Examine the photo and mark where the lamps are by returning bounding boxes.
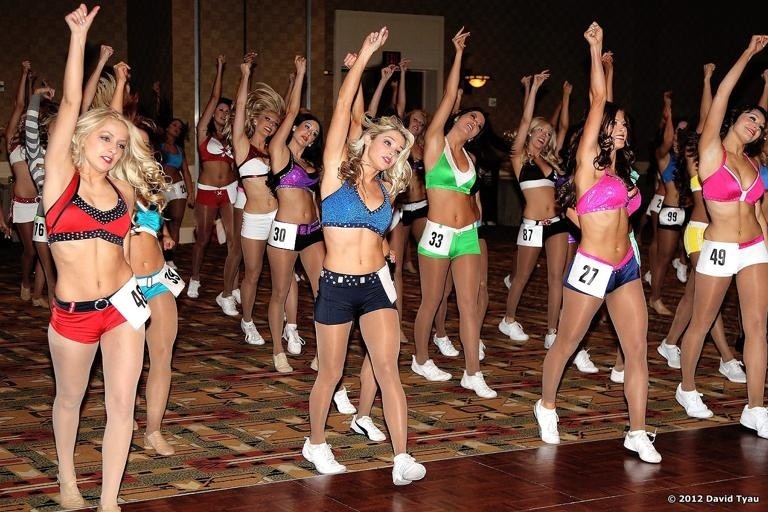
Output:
[465,67,490,89]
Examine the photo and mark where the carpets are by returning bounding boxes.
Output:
[1,236,768,511]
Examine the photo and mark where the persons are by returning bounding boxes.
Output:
[0,3,768,512]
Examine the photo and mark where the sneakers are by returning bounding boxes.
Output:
[460,369,499,400]
[231,288,242,305]
[609,367,624,386]
[644,271,652,284]
[714,356,750,386]
[310,357,320,372]
[504,274,512,290]
[496,316,530,341]
[571,348,600,375]
[298,435,348,477]
[187,277,202,298]
[674,380,716,420]
[399,328,409,344]
[271,349,295,375]
[623,429,666,466]
[350,413,388,444]
[433,333,461,357]
[671,256,689,285]
[141,431,177,458]
[331,385,358,416]
[410,354,452,382]
[239,316,267,346]
[389,451,428,487]
[543,331,558,348]
[477,338,487,362]
[656,337,682,370]
[18,281,31,301]
[166,260,178,271]
[282,322,303,355]
[738,403,768,440]
[530,396,565,446]
[646,297,672,317]
[57,473,85,509]
[30,294,49,310]
[216,290,240,316]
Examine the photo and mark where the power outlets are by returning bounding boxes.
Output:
[488,98,497,107]
